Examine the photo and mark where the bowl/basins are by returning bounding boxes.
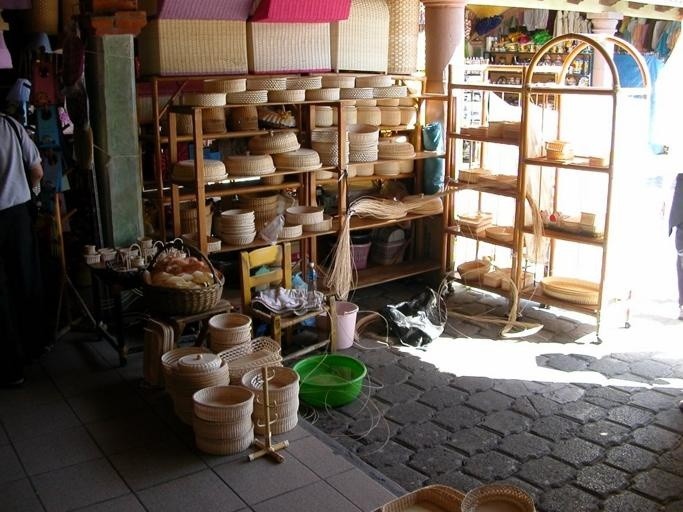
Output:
[292,355,366,408]
[330,229,372,244]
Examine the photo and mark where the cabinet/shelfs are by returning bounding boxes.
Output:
[447,32,651,343]
[145,28,458,318]
[482,43,594,106]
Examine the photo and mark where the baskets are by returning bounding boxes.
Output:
[460,121,521,139]
[546,141,608,167]
[456,210,514,241]
[540,276,599,305]
[560,212,594,237]
[83,235,158,266]
[140,244,225,317]
[171,75,416,182]
[160,312,300,456]
[457,260,534,292]
[169,194,335,252]
[458,167,517,190]
[370,483,537,512]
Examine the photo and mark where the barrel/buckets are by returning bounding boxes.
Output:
[319,300,359,350]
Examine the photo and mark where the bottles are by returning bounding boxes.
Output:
[543,40,589,76]
[316,184,324,207]
[498,56,506,65]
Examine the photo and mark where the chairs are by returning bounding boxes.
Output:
[238,242,336,363]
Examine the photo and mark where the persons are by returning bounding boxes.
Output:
[667,172,682,319]
[1,112,51,386]
